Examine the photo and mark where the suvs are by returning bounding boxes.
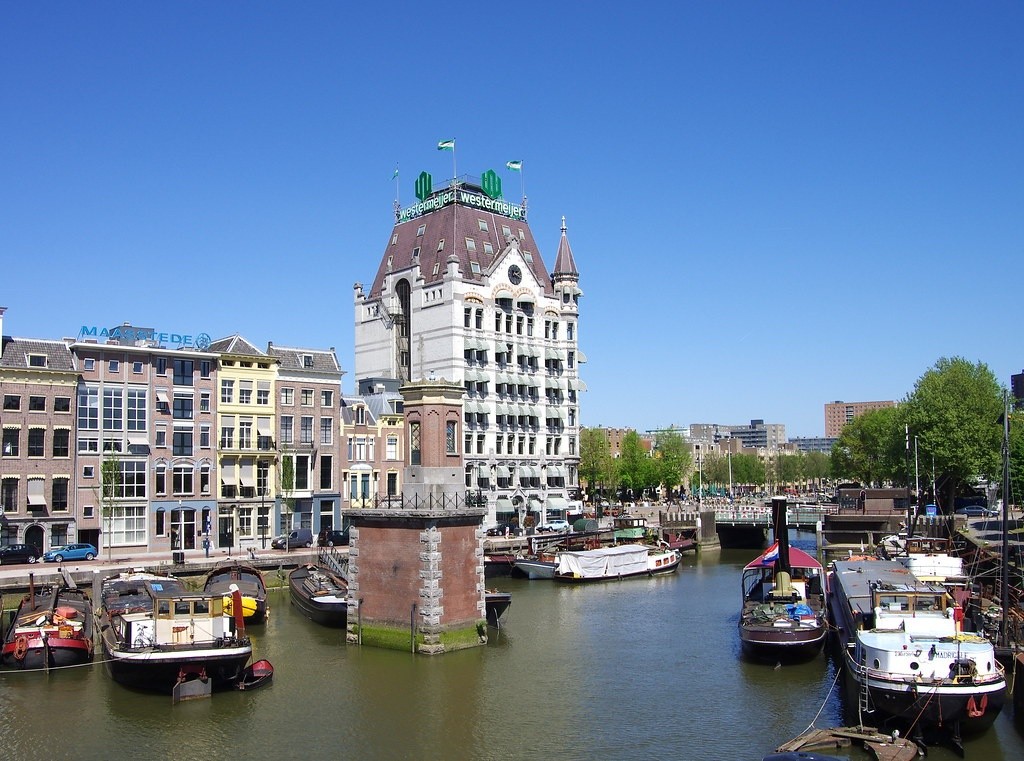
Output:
[544,520,569,532]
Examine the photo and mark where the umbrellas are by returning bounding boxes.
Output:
[690,481,725,499]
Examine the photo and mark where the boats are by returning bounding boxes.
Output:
[237,659,274,692]
[738,496,830,663]
[204,560,267,625]
[289,562,348,628]
[483,537,682,583]
[825,389,1024,759]
[94,561,252,693]
[1,572,94,674]
[485,587,512,629]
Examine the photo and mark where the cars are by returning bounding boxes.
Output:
[317,529,349,547]
[486,523,515,536]
[955,506,999,517]
[0,544,40,565]
[43,543,97,562]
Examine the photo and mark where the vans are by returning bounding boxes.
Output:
[271,529,313,549]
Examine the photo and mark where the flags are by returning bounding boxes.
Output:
[507,160,521,171]
[437,139,453,150]
[762,542,779,565]
[393,166,399,176]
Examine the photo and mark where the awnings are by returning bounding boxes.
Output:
[519,466,532,478]
[529,467,542,477]
[480,466,492,478]
[556,466,566,477]
[529,500,542,512]
[546,466,560,477]
[546,497,569,510]
[496,500,514,512]
[27,495,47,506]
[221,477,237,486]
[240,477,256,487]
[496,466,510,478]
